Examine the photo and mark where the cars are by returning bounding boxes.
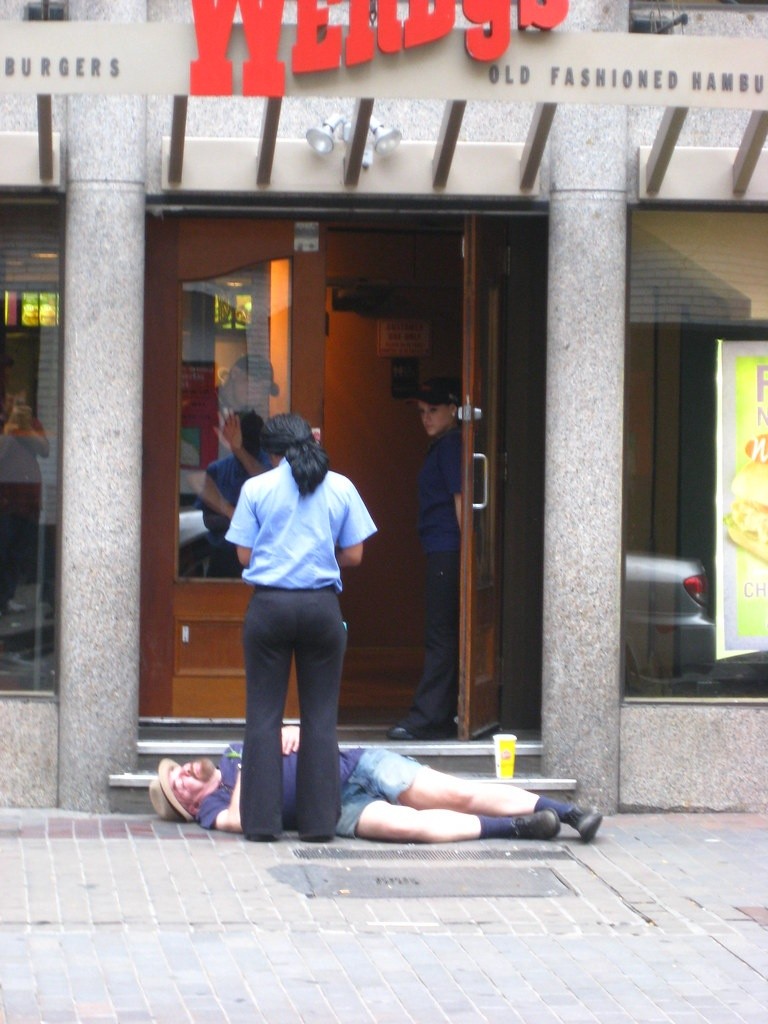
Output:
[624,550,716,679]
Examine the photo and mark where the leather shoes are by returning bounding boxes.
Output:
[564,806,602,842]
[513,808,560,840]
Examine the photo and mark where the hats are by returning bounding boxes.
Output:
[409,377,462,407]
[149,757,194,823]
[235,354,279,397]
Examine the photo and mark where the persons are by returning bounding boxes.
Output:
[0,373,50,612]
[149,726,602,843]
[180,356,280,520]
[386,378,463,738]
[194,410,273,579]
[225,412,377,843]
